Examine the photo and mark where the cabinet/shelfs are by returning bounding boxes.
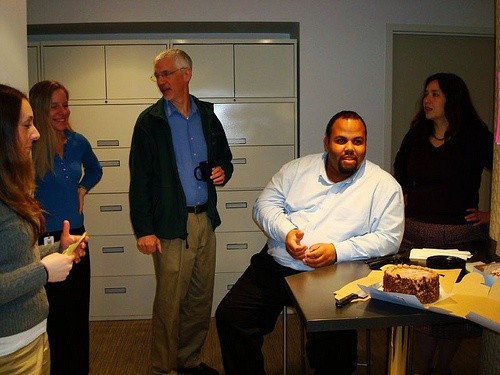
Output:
[168,37,300,320]
[27,38,170,321]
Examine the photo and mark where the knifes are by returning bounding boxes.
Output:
[334,282,382,308]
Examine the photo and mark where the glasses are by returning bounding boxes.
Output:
[150,67,189,82]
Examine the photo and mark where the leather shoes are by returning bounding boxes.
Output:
[177,361,221,375]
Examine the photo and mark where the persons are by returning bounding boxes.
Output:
[214,109,407,375]
[0,82,91,374]
[127,48,235,374]
[29,80,104,375]
[393,72,494,375]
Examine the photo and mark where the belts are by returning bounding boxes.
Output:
[186,203,208,215]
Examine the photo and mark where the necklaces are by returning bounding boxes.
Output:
[432,134,445,141]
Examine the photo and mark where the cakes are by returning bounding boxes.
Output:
[382,264,439,304]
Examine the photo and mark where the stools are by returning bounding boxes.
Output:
[283,306,371,375]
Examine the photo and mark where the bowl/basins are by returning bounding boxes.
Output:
[425,255,465,283]
